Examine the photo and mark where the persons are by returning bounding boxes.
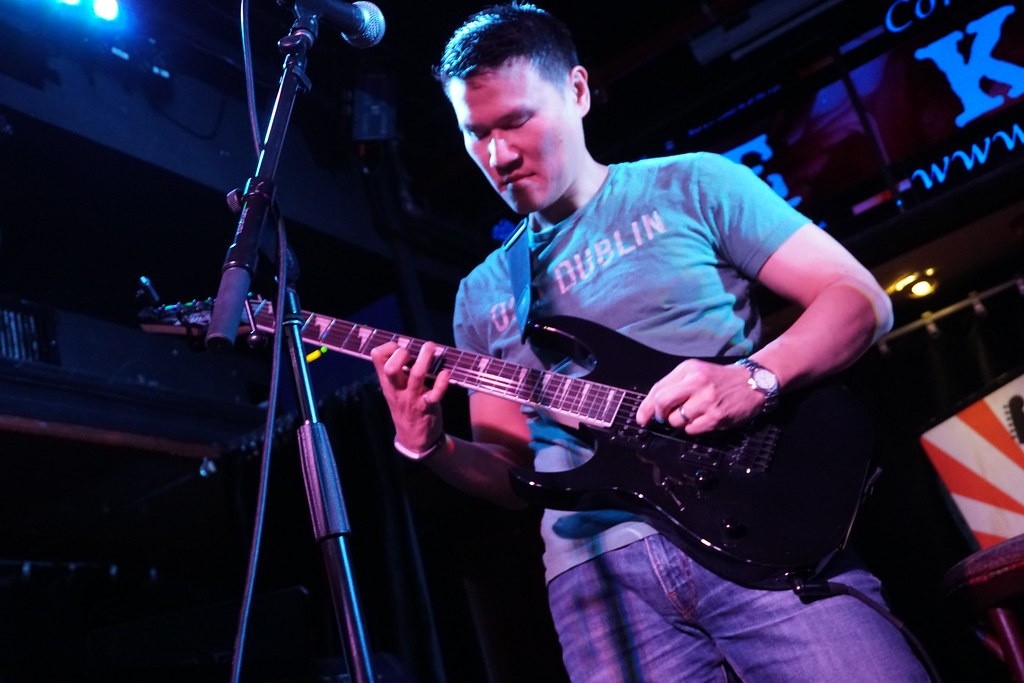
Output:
[369,0,934,683]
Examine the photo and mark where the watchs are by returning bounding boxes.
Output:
[734,356,781,415]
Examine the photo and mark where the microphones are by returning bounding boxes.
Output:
[287,0,385,48]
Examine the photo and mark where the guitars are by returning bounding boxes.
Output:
[133,288,893,593]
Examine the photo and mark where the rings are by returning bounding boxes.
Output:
[679,406,691,423]
